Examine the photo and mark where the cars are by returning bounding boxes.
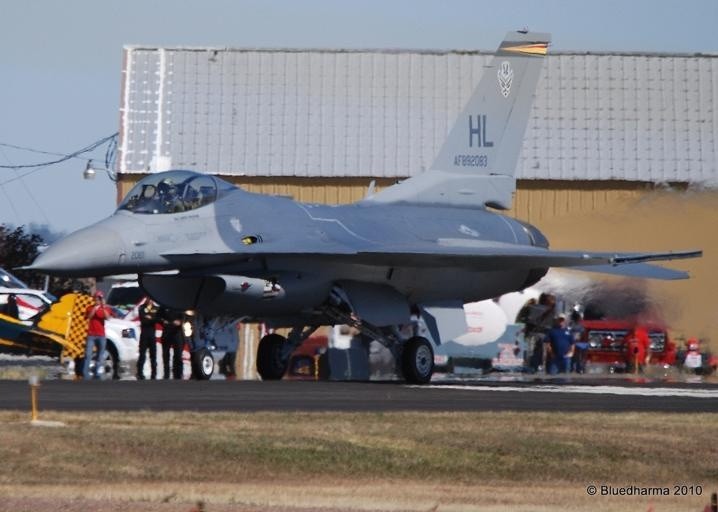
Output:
[0,269,194,380]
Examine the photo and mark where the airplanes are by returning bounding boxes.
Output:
[7,26,702,385]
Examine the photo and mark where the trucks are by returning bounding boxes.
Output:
[567,286,674,370]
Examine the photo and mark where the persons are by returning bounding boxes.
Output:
[134,298,161,381]
[621,325,652,374]
[156,304,187,379]
[83,291,113,381]
[542,313,576,375]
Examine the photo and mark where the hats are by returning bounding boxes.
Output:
[92,290,105,298]
